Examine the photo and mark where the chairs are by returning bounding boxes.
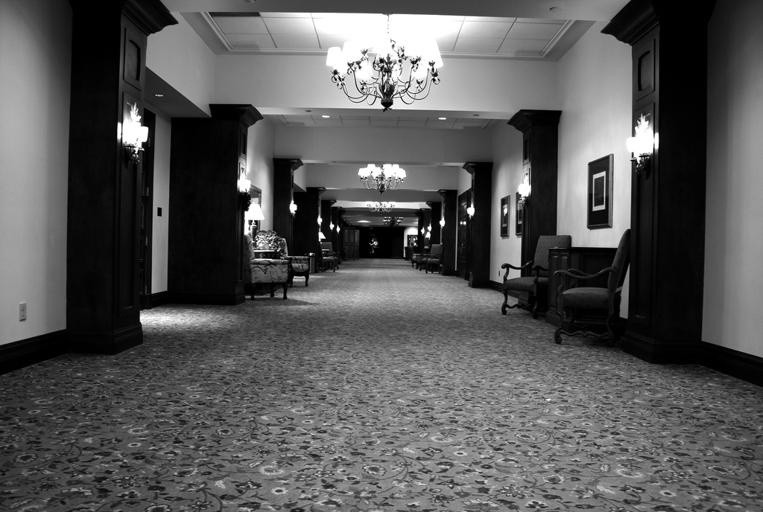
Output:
[241,235,289,300]
[319,242,338,272]
[410,242,443,274]
[254,229,310,287]
[550,227,634,347]
[499,232,572,320]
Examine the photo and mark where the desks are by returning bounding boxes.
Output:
[546,245,618,327]
[251,248,280,298]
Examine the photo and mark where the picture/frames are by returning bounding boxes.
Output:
[585,154,615,230]
[247,184,262,237]
[514,190,524,236]
[499,194,510,238]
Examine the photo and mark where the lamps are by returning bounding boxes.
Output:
[318,231,326,242]
[382,215,404,226]
[320,11,446,111]
[355,162,406,196]
[244,201,265,250]
[628,110,656,174]
[123,99,149,161]
[366,191,394,215]
[236,169,250,196]
[517,162,531,198]
